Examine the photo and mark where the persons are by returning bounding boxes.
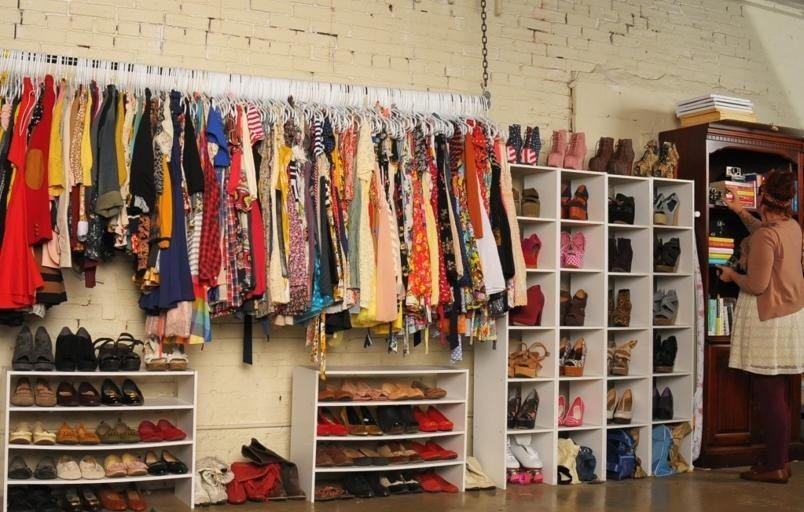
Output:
[715,170,804,482]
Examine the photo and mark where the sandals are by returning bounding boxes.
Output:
[507,232,682,430]
[12,325,189,372]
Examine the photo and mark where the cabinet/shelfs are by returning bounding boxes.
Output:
[473,159,697,491]
[289,364,470,503]
[658,118,803,467]
[3,368,198,512]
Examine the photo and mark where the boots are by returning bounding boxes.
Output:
[193,437,306,506]
[464,455,496,491]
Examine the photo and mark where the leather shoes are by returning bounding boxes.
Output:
[740,463,792,484]
[8,376,189,512]
[315,378,459,500]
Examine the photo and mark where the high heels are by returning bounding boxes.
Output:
[506,123,682,225]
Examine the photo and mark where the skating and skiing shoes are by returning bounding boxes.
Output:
[507,434,543,484]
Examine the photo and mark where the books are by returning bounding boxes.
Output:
[708,166,799,337]
